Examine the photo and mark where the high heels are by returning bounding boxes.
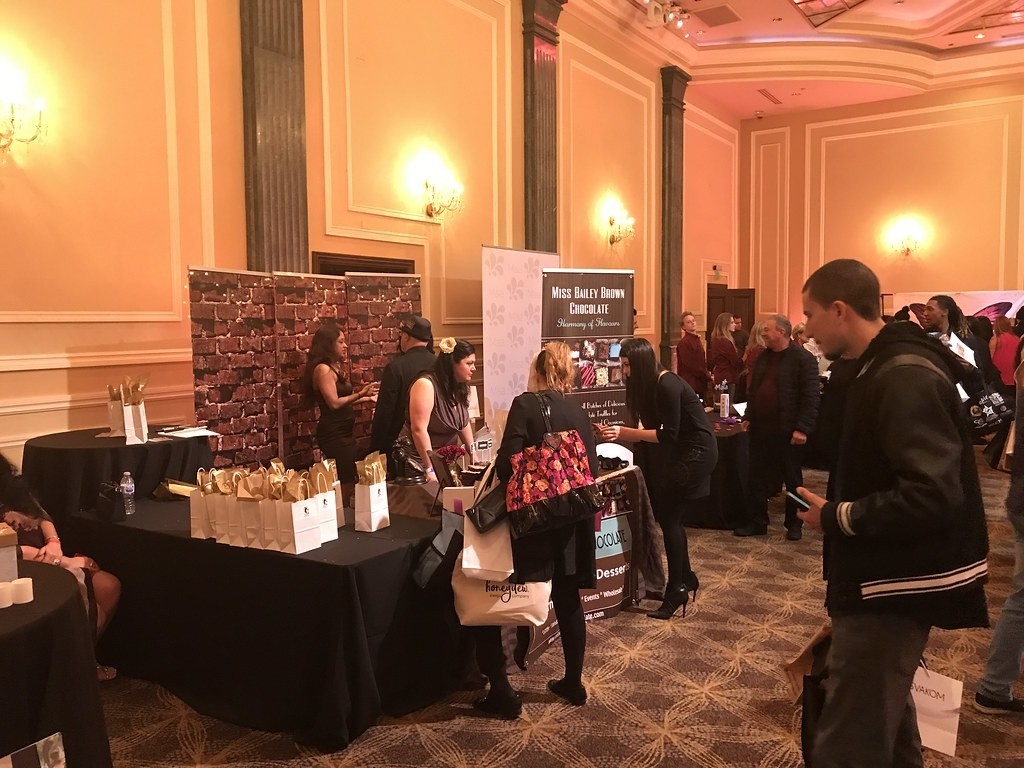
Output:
[682,568,700,602]
[647,584,689,620]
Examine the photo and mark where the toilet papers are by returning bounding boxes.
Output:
[0,577,35,609]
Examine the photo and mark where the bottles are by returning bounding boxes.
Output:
[120,471,135,516]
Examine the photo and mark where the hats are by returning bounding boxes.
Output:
[398,316,431,342]
[894,306,910,321]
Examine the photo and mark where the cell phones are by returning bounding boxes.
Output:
[786,491,811,511]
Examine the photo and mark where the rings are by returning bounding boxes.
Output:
[90,563,92,567]
[54,559,60,564]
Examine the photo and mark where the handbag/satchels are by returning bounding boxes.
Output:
[784,617,834,707]
[190,465,390,556]
[912,658,963,758]
[965,380,1015,437]
[413,456,553,627]
[506,392,606,539]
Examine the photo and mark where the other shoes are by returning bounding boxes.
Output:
[788,526,804,541]
[973,690,1024,717]
[473,693,522,716]
[734,522,766,536]
[547,678,587,707]
[96,663,117,682]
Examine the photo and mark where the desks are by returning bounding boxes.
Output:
[0,560,112,768]
[23,422,213,512]
[681,410,751,527]
[57,493,471,753]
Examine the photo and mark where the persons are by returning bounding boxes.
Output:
[306,324,379,485]
[634,309,638,329]
[391,336,490,480]
[596,338,718,620]
[974,360,1024,714]
[881,295,1024,471]
[371,316,437,466]
[802,259,991,768]
[0,451,121,681]
[733,315,820,540]
[676,312,832,405]
[469,341,600,717]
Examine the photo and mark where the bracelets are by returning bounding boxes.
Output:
[357,392,361,399]
[425,468,434,473]
[45,536,61,544]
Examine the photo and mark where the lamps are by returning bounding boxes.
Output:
[401,147,462,218]
[0,61,44,148]
[592,192,635,249]
[881,216,929,259]
